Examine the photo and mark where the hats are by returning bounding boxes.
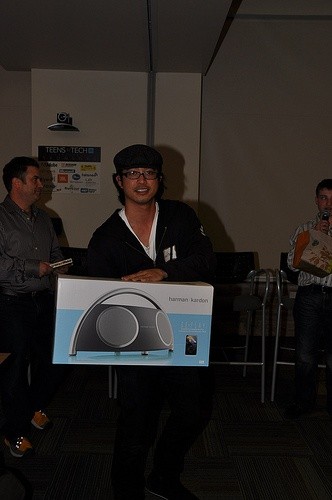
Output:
[113,145,163,172]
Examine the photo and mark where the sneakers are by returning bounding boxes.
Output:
[31,406,51,430]
[4,432,34,457]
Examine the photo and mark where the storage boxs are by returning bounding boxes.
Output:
[53,274,214,367]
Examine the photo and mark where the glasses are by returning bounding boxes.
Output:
[121,171,158,180]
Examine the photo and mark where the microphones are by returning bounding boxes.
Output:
[322,210,329,221]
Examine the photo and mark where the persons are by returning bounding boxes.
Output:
[85,146,215,500]
[0,156,73,458]
[285,179,332,417]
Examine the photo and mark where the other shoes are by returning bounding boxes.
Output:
[146,464,199,500]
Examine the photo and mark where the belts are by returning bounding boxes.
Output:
[298,284,332,295]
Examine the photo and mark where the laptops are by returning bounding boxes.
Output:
[211,252,255,285]
[281,252,300,284]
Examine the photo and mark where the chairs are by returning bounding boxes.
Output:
[209,252,269,404]
[270,252,327,402]
